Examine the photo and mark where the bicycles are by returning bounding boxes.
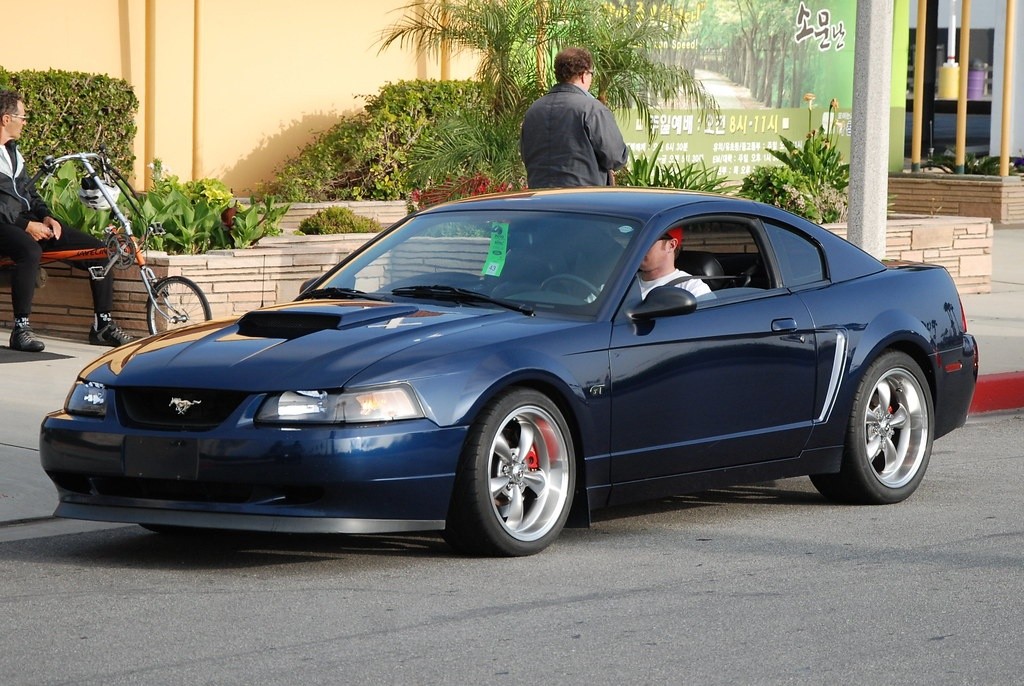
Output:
[0,145,211,336]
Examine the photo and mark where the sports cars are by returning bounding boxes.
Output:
[39,185,982,561]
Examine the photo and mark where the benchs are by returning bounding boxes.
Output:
[713,252,769,289]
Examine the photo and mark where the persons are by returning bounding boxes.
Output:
[583,225,717,306]
[0,89,141,352]
[520,47,629,190]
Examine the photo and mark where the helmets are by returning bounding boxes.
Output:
[79,177,121,210]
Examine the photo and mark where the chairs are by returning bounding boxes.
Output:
[504,248,572,295]
[675,253,736,291]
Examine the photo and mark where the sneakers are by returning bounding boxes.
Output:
[89,321,143,347]
[10,326,45,351]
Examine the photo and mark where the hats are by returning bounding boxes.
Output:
[666,226,683,247]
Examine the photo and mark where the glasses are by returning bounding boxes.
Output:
[9,114,30,122]
